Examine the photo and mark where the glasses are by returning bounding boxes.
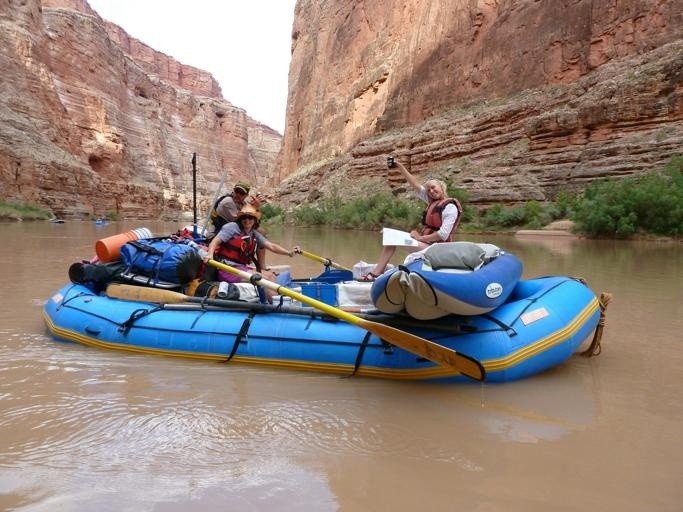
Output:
[235,188,245,196]
[241,215,253,220]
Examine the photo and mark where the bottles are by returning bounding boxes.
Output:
[218,282,228,298]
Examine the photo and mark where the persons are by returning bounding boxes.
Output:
[357,156,463,282]
[201,204,302,300]
[209,179,279,277]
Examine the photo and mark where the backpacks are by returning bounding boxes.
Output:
[119,236,202,285]
[184,277,241,302]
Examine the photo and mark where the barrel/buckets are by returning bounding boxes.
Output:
[95,227,154,263]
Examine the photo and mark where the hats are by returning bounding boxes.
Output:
[236,203,262,221]
[232,180,251,194]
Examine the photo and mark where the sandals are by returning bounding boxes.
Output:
[356,272,379,283]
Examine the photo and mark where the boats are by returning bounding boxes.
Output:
[49,219,64,223]
[42,260,603,381]
[95,219,105,225]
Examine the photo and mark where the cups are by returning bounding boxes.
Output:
[387,157,394,169]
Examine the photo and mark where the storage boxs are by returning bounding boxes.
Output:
[300,280,337,307]
[337,280,377,307]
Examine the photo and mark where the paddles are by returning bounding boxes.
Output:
[209,258,486,381]
